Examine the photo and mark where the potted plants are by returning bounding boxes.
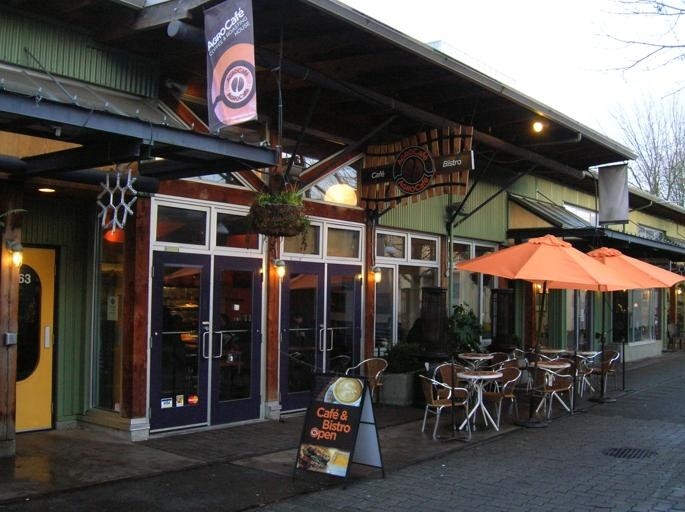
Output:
[377,340,425,407]
[249,188,310,238]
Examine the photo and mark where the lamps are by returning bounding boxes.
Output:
[272,258,286,278]
[4,239,26,266]
[369,264,382,283]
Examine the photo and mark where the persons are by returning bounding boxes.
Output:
[159,300,319,392]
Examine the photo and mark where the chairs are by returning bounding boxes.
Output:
[344,357,389,404]
[417,345,620,439]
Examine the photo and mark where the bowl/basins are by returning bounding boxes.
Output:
[333,377,364,406]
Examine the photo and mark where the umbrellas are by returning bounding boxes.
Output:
[455,232,619,417]
[539,246,685,401]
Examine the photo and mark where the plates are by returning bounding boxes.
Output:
[324,383,361,408]
